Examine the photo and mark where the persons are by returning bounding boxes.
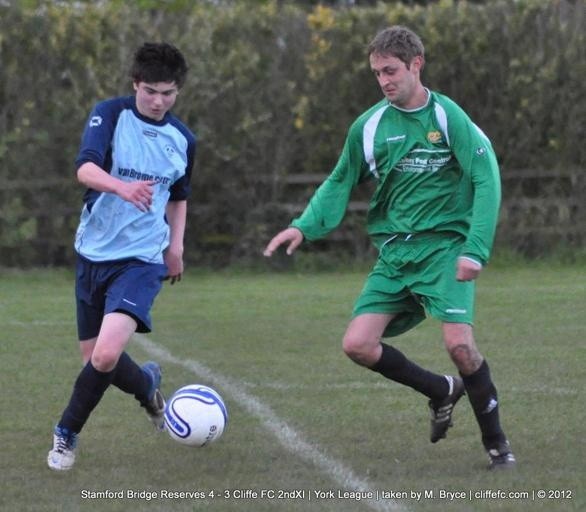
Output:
[258,21,516,472]
[42,39,195,472]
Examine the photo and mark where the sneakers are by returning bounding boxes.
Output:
[139,361,167,433]
[482,432,516,472]
[46,422,78,472]
[429,374,465,443]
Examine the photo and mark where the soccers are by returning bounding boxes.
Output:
[163,385,227,448]
